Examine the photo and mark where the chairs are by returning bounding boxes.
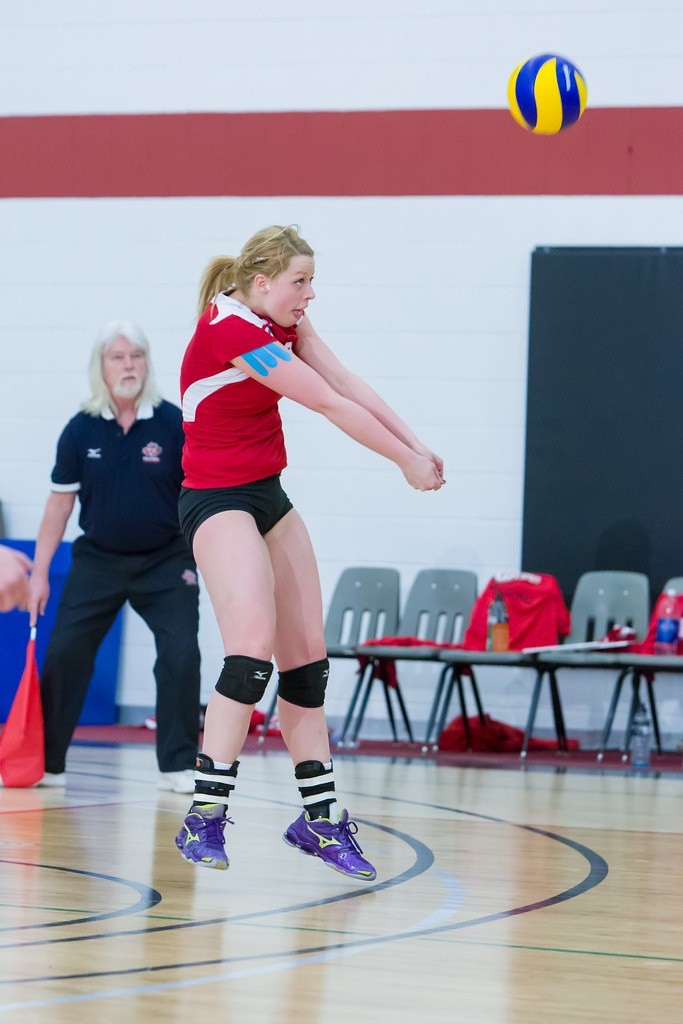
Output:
[431,572,569,756]
[349,570,489,751]
[517,569,659,763]
[256,567,416,748]
[620,577,682,761]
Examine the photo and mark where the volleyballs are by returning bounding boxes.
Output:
[506,53,589,135]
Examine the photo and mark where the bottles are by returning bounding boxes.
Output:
[631,704,652,769]
[655,589,679,655]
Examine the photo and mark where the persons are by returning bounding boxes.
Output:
[175,223,447,881]
[26,318,201,794]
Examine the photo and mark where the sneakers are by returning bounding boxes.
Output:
[33,773,67,787]
[174,803,235,871]
[158,770,196,793]
[282,808,377,881]
